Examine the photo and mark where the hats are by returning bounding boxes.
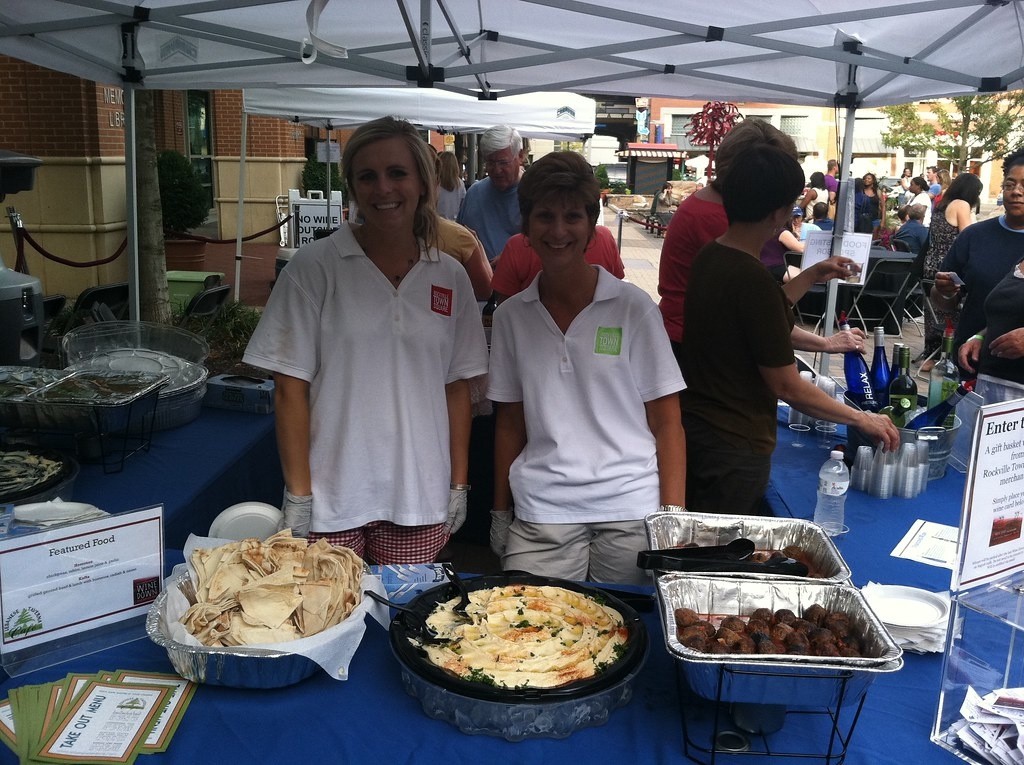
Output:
[792,207,803,216]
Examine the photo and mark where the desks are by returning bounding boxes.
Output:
[0,386,1024,765]
[843,249,917,332]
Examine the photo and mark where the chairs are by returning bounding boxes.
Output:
[782,237,925,339]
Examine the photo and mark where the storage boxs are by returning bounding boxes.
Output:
[166,270,225,316]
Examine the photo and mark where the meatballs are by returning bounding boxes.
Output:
[675,604,867,658]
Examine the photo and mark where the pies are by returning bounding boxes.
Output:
[180,527,365,649]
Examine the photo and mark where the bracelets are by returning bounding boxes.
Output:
[940,293,958,300]
[450,483,470,491]
[660,503,687,511]
[966,335,983,342]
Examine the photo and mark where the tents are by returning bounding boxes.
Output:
[0,0,1024,393]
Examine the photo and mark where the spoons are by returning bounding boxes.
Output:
[364,590,451,643]
[442,563,478,615]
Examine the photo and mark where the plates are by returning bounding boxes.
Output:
[207,501,280,541]
[864,583,949,629]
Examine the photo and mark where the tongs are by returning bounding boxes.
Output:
[637,539,809,579]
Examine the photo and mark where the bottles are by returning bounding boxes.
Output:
[927,319,959,430]
[889,347,917,410]
[870,327,891,408]
[603,194,608,207]
[878,398,910,428]
[891,342,904,380]
[838,311,879,412]
[482,297,495,327]
[904,380,976,431]
[813,450,849,536]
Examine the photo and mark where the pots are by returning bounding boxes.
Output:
[388,567,651,739]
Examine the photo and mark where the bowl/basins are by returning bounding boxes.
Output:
[63,354,209,430]
[0,443,79,505]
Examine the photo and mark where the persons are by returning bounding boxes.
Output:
[242,116,488,566]
[416,144,494,303]
[929,144,1024,390]
[892,162,983,373]
[488,150,688,590]
[425,150,466,223]
[657,117,900,512]
[455,124,530,274]
[485,225,626,329]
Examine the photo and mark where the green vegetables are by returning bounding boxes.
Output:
[412,586,635,688]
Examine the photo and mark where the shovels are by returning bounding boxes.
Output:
[443,563,478,618]
[365,590,450,644]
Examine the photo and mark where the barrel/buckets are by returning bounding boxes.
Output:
[843,391,962,480]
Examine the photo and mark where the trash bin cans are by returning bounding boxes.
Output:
[167,271,225,326]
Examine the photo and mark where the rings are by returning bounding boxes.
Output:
[856,345,858,350]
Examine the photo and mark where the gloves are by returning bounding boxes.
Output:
[278,492,313,538]
[489,508,513,556]
[443,489,467,534]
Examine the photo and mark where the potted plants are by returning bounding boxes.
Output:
[595,162,611,199]
[303,154,349,223]
[157,148,212,273]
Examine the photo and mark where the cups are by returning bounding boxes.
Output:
[851,440,930,499]
[844,263,864,283]
[788,371,837,449]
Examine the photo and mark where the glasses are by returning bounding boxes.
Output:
[486,155,516,168]
[1000,184,1024,193]
